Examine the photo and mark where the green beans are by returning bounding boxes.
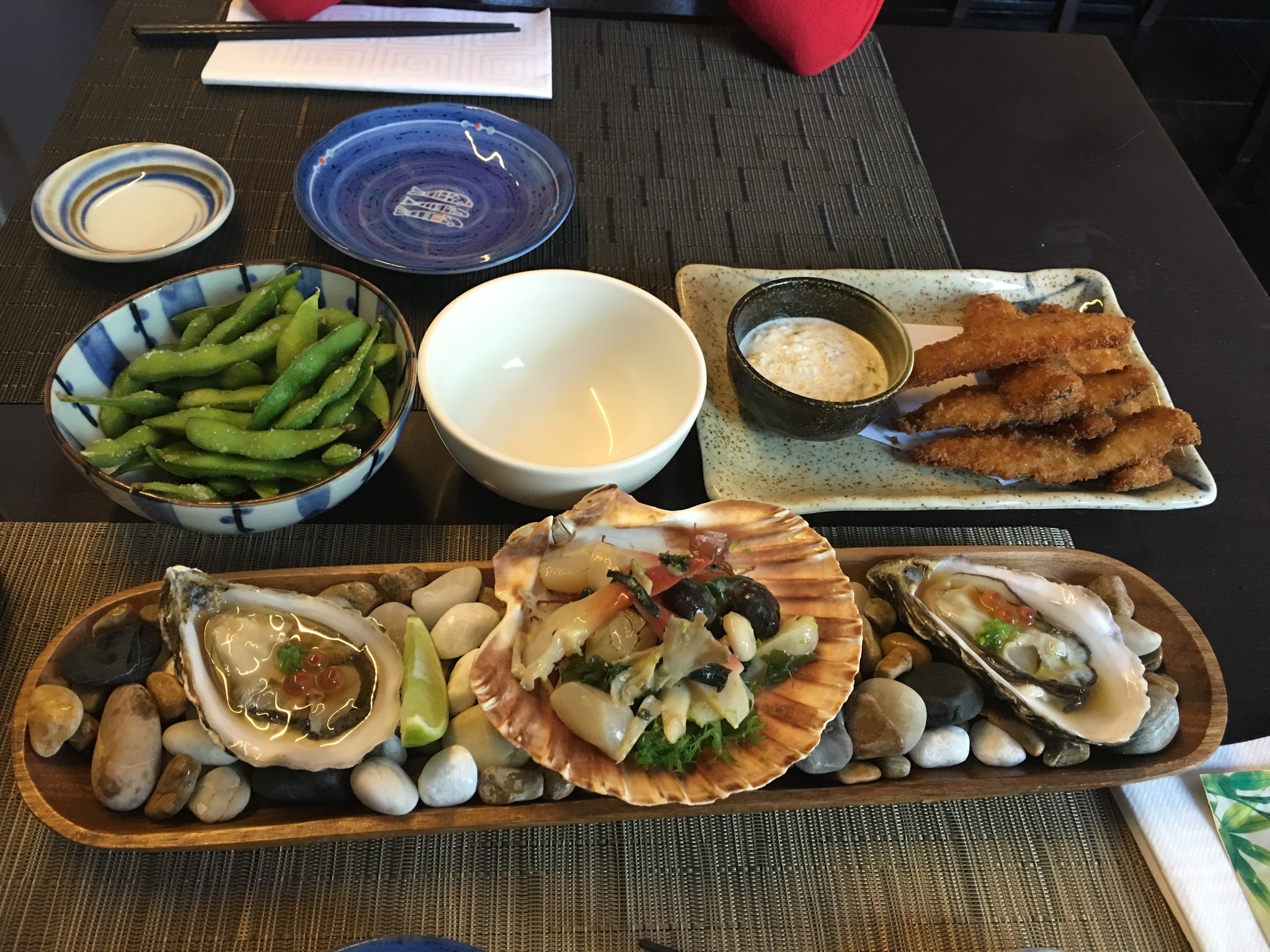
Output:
[57,270,400,502]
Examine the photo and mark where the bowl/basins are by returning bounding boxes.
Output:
[727,276,914,440]
[418,269,706,512]
[43,258,419,535]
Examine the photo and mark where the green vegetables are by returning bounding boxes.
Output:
[562,553,817,775]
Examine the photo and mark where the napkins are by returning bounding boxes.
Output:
[201,0,553,100]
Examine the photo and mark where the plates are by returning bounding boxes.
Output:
[291,101,576,275]
[676,263,1217,516]
[30,141,234,263]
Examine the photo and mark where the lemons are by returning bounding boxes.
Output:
[400,615,449,746]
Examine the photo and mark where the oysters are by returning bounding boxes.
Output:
[866,555,1152,746]
[159,565,406,773]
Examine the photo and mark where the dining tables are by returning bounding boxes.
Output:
[0,0,1270,952]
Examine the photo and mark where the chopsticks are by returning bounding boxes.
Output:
[130,20,520,40]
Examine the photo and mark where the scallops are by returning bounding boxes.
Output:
[469,482,863,809]
